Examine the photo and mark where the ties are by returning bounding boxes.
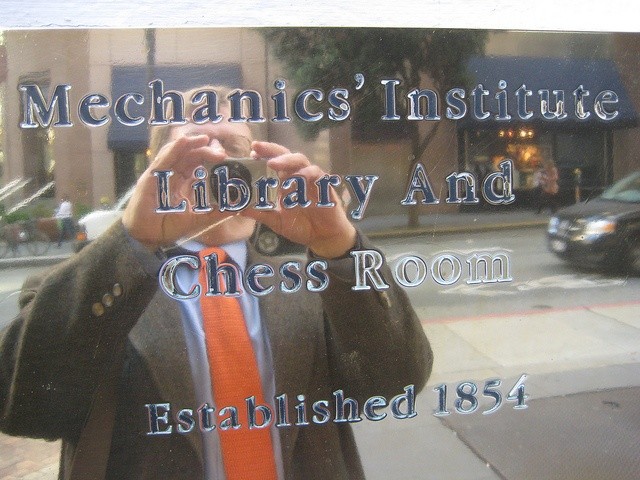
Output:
[196,246,276,476]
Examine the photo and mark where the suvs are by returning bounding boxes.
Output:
[72,185,135,253]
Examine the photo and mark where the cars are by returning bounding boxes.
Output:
[547,169,640,278]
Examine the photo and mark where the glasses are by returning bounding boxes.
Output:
[183,132,255,158]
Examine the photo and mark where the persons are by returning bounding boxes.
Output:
[1,85,434,479]
[535,160,560,215]
[54,195,77,249]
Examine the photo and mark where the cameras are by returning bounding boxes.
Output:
[202,158,270,208]
